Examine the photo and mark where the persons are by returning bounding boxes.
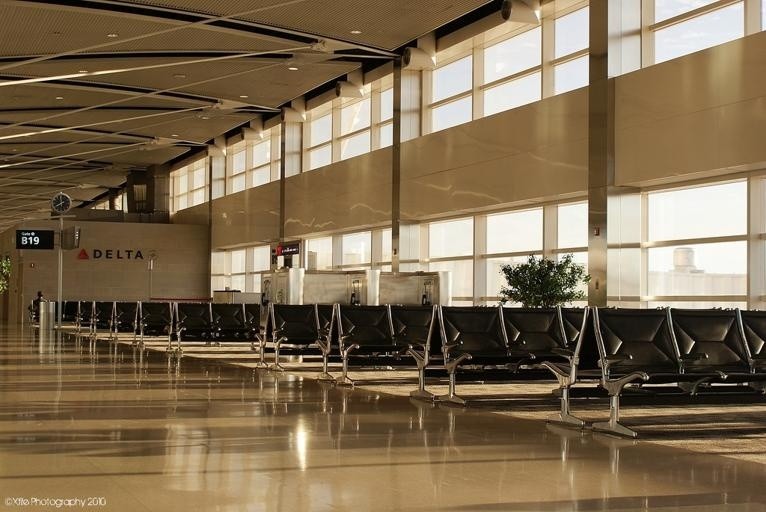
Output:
[28,291,49,318]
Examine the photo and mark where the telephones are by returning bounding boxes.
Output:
[261,292,267,306]
[422,294,426,303]
[350,292,355,305]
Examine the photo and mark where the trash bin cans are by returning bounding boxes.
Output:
[40,331,55,364]
[40,302,55,331]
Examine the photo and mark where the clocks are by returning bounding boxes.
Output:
[52,193,71,214]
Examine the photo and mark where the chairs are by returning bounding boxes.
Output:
[62,301,765,441]
